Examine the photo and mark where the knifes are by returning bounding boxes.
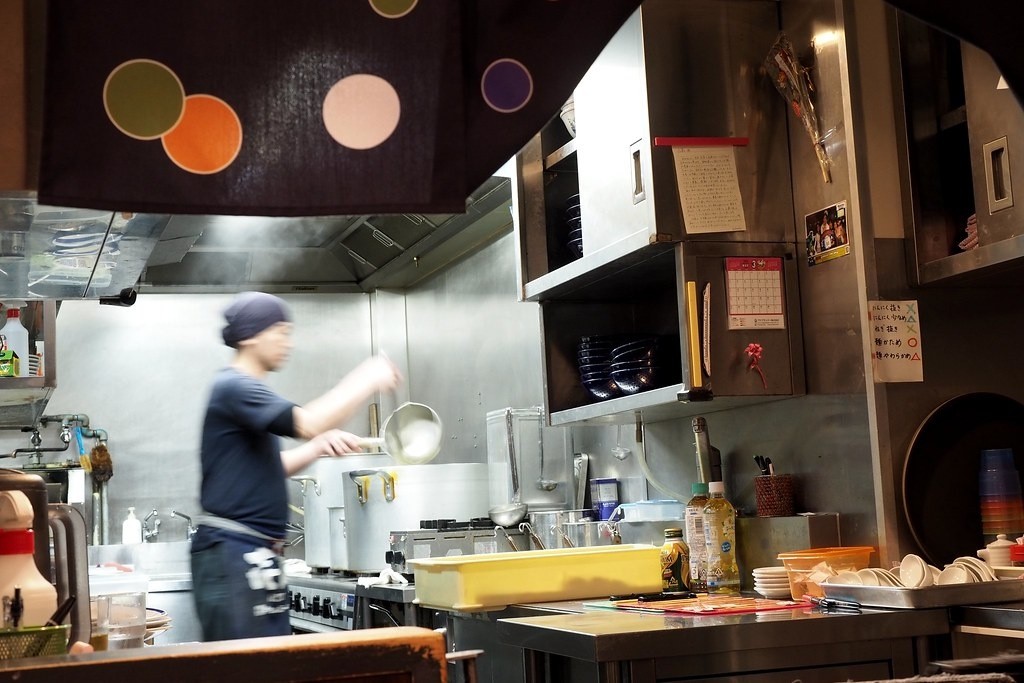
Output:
[608,591,689,601]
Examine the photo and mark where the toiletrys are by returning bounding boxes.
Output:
[122,506,142,544]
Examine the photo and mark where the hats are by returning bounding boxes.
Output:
[223,292,294,342]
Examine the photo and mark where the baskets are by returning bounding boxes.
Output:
[0,624,72,660]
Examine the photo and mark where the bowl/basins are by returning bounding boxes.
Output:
[564,193,583,259]
[824,554,998,588]
[575,334,655,399]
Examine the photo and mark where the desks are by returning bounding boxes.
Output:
[495,599,1024,683]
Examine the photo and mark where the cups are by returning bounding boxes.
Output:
[97,592,147,651]
[979,447,1024,547]
[977,534,1018,568]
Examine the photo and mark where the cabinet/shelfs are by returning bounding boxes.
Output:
[884,0,1024,289]
[511,0,805,428]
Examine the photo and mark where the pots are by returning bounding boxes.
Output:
[328,506,355,572]
[342,463,490,575]
[291,452,394,568]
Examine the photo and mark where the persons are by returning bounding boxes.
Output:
[810,210,846,255]
[189,289,402,640]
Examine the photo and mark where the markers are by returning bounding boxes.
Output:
[801,593,861,609]
[753,454,774,477]
[636,592,690,603]
[3,583,76,657]
[609,591,664,601]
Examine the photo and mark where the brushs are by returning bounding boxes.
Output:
[91,443,113,482]
[73,426,92,474]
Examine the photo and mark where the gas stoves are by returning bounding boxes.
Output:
[283,566,385,633]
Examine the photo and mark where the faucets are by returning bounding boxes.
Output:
[170,510,194,541]
[143,508,158,542]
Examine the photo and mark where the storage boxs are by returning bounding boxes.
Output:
[406,543,664,613]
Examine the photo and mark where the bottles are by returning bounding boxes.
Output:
[702,482,740,594]
[660,528,690,597]
[684,483,712,593]
[0,301,29,376]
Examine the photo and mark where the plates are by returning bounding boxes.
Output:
[993,567,1024,580]
[90,604,174,642]
[28,354,40,376]
[752,566,792,599]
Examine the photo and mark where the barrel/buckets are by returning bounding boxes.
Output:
[562,521,615,550]
[528,508,595,554]
